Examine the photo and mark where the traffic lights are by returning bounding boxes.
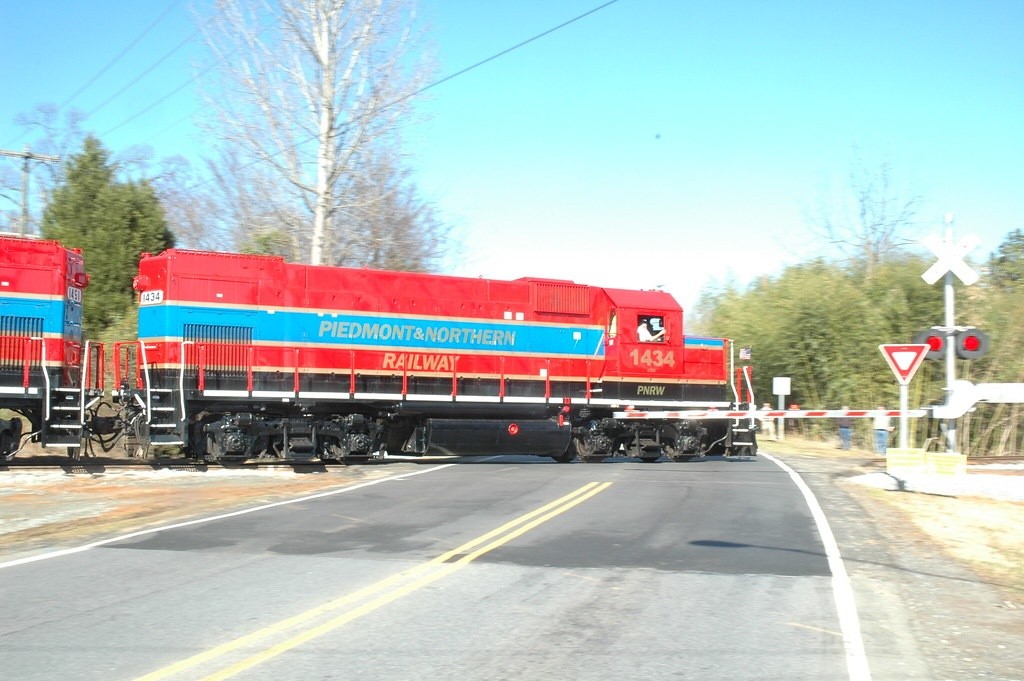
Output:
[918,329,946,359]
[957,329,989,360]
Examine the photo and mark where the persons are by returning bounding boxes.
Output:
[638,319,665,342]
[760,403,775,437]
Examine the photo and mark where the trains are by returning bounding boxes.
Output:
[0,233,758,471]
